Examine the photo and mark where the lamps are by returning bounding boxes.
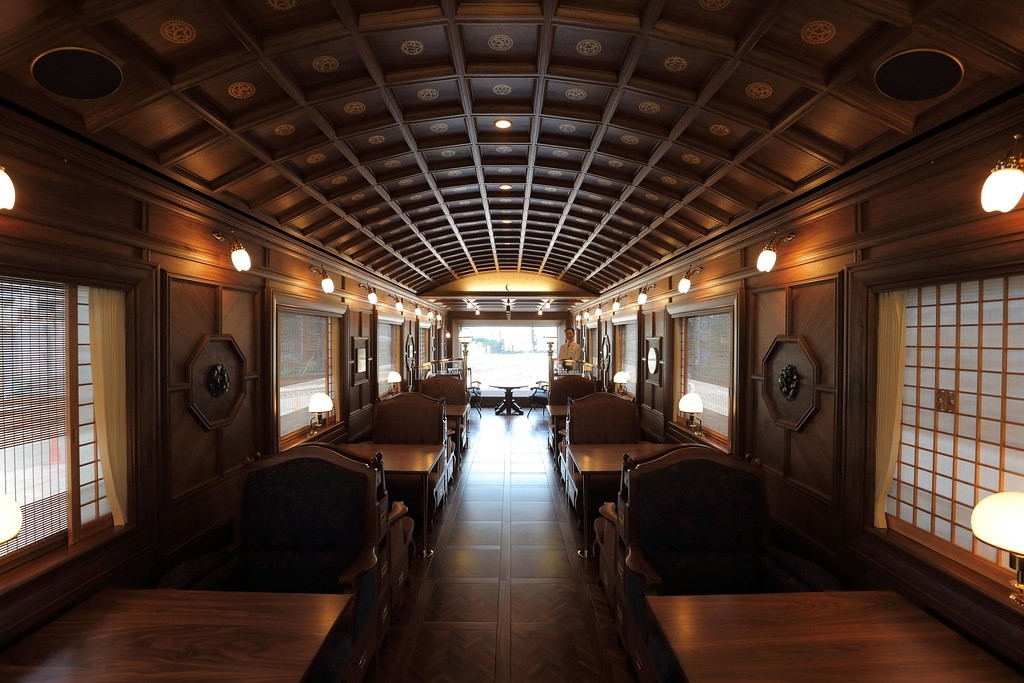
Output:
[636,281,655,305]
[386,370,402,395]
[307,391,335,436]
[679,392,707,435]
[0,491,24,546]
[574,301,607,322]
[501,299,516,316]
[969,490,1024,589]
[675,262,702,293]
[979,134,1024,215]
[213,229,253,274]
[358,282,378,304]
[462,299,482,316]
[537,298,556,316]
[410,301,421,316]
[428,309,452,340]
[614,371,629,396]
[612,295,627,311]
[753,230,799,274]
[309,264,336,295]
[0,162,18,212]
[388,294,404,311]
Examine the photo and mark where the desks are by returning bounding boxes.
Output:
[345,440,446,559]
[639,590,1020,683]
[447,402,468,472]
[563,444,674,560]
[488,383,529,416]
[0,582,360,683]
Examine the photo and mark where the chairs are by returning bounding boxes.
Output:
[556,396,569,496]
[567,394,652,535]
[156,444,396,683]
[377,394,447,529]
[418,376,469,451]
[612,448,844,683]
[546,402,568,471]
[590,439,755,608]
[547,374,598,461]
[241,441,417,619]
[527,381,548,418]
[466,381,483,418]
[378,392,457,498]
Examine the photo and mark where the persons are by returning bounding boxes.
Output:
[559,328,581,372]
